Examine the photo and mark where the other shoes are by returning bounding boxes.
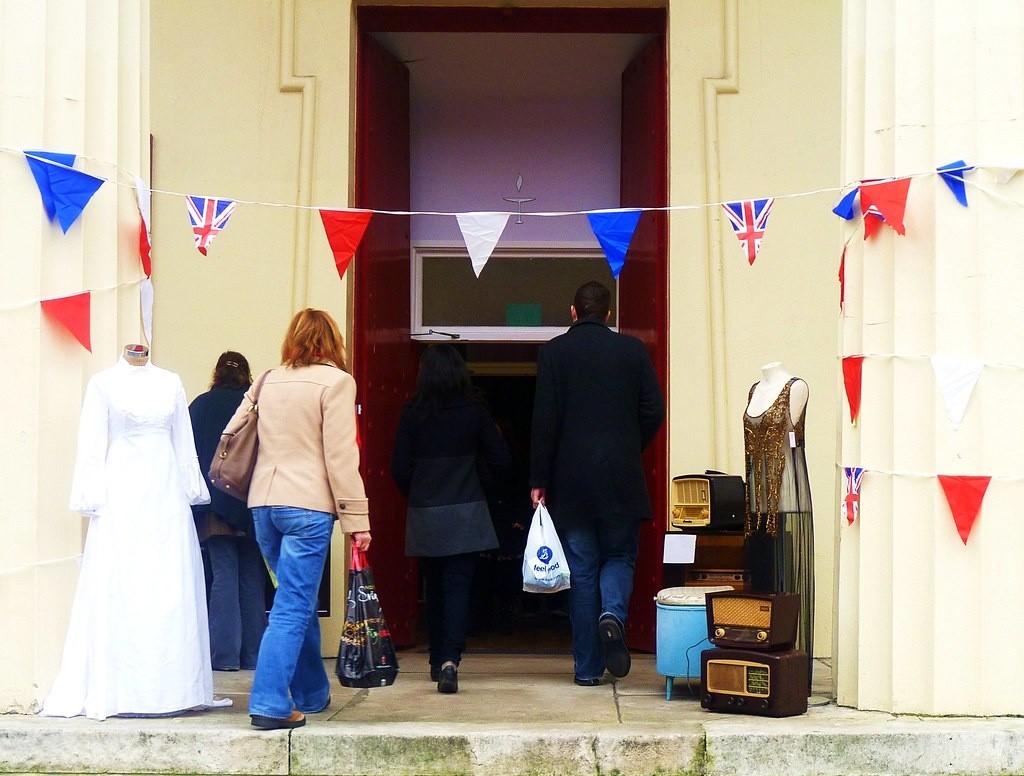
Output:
[574,675,599,686]
[251,711,306,728]
[437,666,459,694]
[599,615,632,677]
[430,664,441,681]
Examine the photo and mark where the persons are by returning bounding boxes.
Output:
[187,350,266,670]
[391,346,511,694]
[47,344,213,719]
[529,280,664,685]
[222,308,371,726]
[742,362,813,697]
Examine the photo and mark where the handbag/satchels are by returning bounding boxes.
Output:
[522,502,571,593]
[335,541,398,689]
[207,367,271,503]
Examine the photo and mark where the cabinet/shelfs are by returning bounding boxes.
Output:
[662,530,775,596]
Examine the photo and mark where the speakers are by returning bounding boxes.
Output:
[669,474,747,529]
[704,589,800,651]
[700,647,810,717]
